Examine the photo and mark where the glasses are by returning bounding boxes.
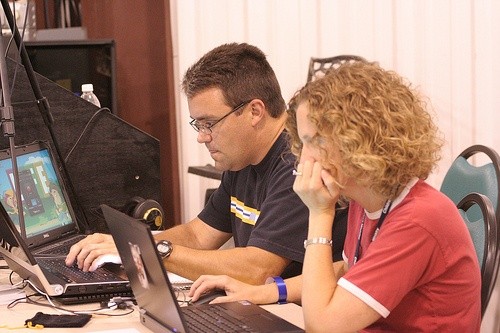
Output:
[189,102,246,135]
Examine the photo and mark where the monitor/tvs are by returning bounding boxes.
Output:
[23,39,117,116]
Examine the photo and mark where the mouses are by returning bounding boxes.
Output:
[186,291,228,306]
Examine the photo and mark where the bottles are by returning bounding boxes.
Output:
[81,84,101,109]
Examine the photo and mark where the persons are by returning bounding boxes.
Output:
[187,60,483,332]
[63,42,350,286]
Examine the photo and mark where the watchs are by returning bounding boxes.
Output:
[155,239,173,260]
[303,237,333,248]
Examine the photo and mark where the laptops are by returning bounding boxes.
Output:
[99,204,307,333]
[0,139,94,257]
[0,202,196,305]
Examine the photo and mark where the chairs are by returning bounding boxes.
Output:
[441,143,500,308]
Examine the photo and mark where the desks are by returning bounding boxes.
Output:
[1,257,306,333]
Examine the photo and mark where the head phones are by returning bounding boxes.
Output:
[119,196,166,232]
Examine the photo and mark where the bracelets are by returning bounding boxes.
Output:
[264,275,288,306]
